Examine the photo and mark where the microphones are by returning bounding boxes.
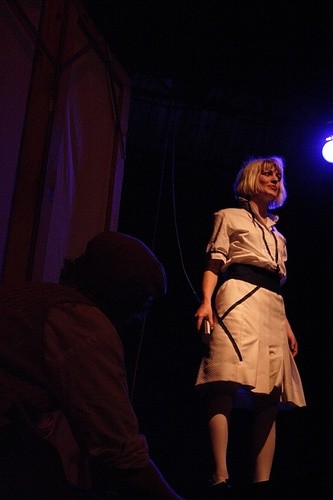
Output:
[201,319,211,340]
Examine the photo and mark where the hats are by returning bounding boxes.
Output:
[66,226,170,302]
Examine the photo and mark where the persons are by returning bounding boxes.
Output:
[192,158,306,495]
[0,233,182,500]
